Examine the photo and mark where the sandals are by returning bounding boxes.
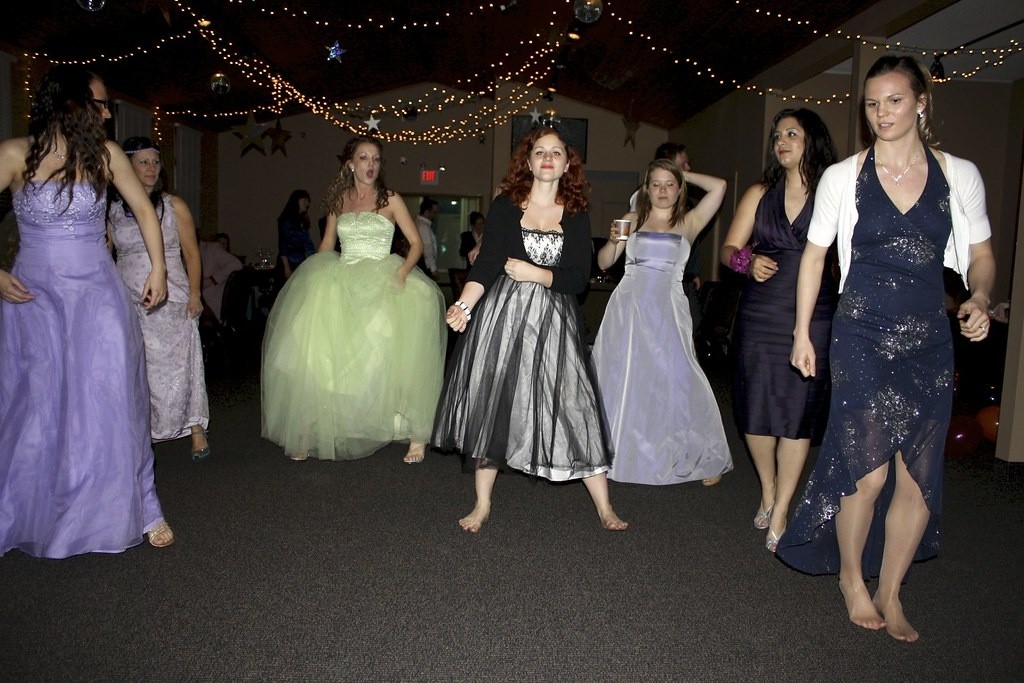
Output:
[405,443,426,463]
[290,451,307,459]
[754,497,776,530]
[149,521,175,547]
[764,510,787,551]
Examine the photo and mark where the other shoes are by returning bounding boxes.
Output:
[191,427,210,463]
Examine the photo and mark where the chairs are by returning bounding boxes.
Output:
[448,267,468,300]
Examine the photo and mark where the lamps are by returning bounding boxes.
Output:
[420,163,446,185]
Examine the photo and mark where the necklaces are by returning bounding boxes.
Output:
[875,153,919,187]
[47,142,66,161]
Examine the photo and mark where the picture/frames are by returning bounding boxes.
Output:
[511,115,588,164]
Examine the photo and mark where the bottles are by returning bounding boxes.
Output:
[246,247,277,270]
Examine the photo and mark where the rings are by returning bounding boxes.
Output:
[980,327,986,332]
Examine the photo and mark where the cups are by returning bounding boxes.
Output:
[614,219,632,240]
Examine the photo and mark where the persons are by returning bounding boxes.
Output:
[719,108,840,553]
[271,190,316,285]
[431,127,628,533]
[179,228,243,335]
[0,62,175,559]
[259,137,448,464]
[590,158,734,486]
[413,199,440,281]
[629,142,704,336]
[776,55,996,642]
[105,136,211,462]
[459,211,485,272]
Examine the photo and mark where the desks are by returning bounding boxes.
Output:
[243,268,276,308]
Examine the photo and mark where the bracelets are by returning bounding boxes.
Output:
[454,301,471,322]
[730,247,753,277]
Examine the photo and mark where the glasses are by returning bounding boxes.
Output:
[93,98,109,109]
